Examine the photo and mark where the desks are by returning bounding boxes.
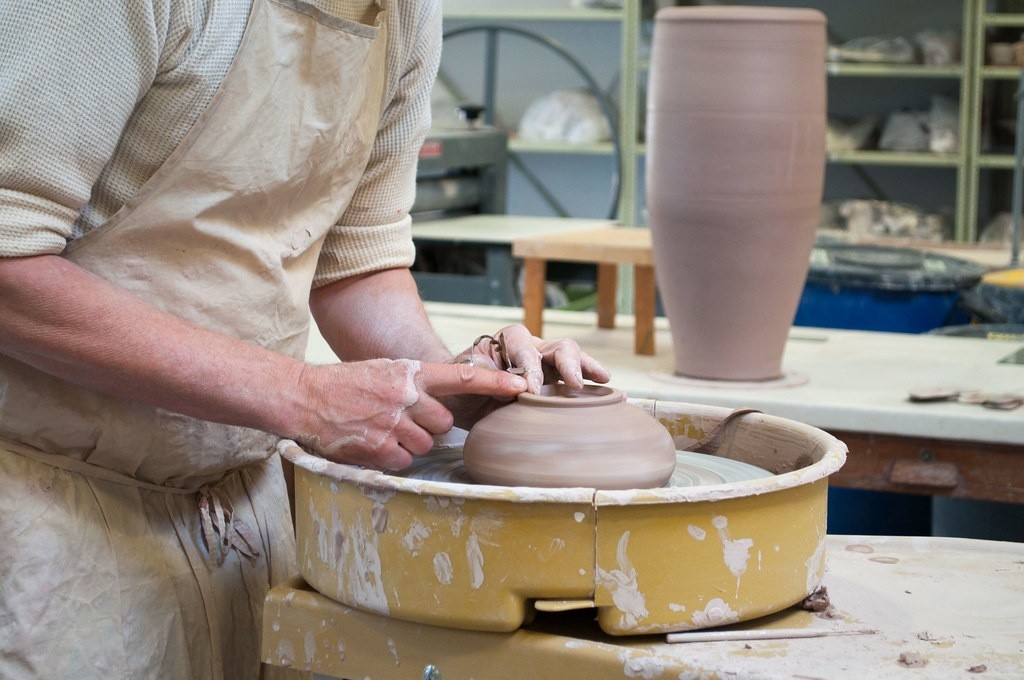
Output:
[304,298,1024,505]
[255,532,1023,679]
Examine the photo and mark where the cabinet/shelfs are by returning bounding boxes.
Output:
[511,229,662,356]
[407,212,629,310]
[436,0,1024,245]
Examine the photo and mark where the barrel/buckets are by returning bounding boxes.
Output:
[929,267,1024,542]
[796,244,990,537]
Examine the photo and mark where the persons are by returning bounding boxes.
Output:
[0,1,611,680]
